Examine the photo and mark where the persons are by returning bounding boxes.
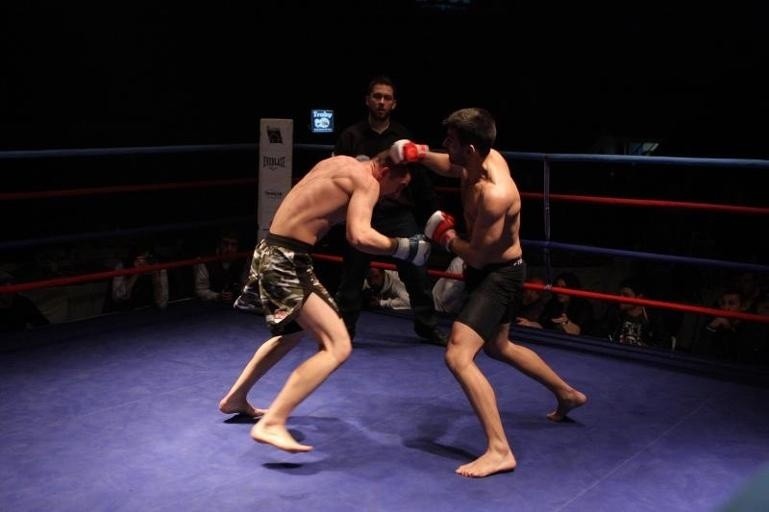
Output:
[749,296,769,367]
[101,244,165,315]
[704,272,769,325]
[560,278,658,349]
[388,106,588,478]
[193,229,251,305]
[332,76,451,346]
[696,288,756,385]
[361,266,412,311]
[219,148,432,455]
[514,274,578,336]
[521,277,545,321]
[1,266,50,332]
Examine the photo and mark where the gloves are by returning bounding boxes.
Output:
[391,139,430,165]
[425,211,458,253]
[393,234,432,266]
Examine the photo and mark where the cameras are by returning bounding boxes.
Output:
[144,255,157,264]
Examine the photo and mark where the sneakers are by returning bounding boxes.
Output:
[415,326,448,346]
